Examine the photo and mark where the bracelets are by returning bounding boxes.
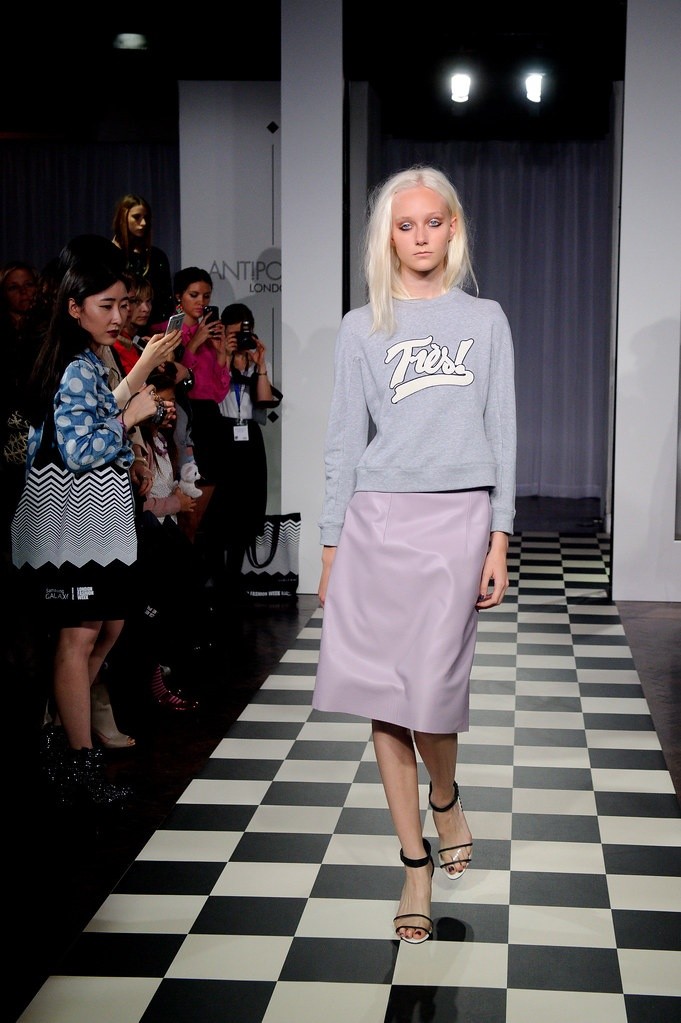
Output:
[183,368,195,386]
[258,371,267,375]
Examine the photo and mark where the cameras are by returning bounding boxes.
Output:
[231,320,258,352]
[148,404,167,426]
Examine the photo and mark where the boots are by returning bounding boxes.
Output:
[70,748,132,809]
[42,721,74,803]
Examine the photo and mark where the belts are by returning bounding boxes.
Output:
[223,417,253,426]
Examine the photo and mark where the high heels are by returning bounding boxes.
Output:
[429,781,473,881]
[394,839,435,944]
[88,682,134,748]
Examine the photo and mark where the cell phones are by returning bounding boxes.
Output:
[165,313,185,336]
[203,306,219,335]
[133,336,147,351]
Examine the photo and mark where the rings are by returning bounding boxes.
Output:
[209,329,212,332]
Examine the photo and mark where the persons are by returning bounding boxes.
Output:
[312,166,517,944]
[1,192,272,765]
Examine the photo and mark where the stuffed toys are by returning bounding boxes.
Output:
[177,461,203,497]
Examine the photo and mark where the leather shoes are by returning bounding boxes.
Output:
[153,691,199,712]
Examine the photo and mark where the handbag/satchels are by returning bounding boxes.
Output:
[10,448,145,619]
[237,513,301,597]
